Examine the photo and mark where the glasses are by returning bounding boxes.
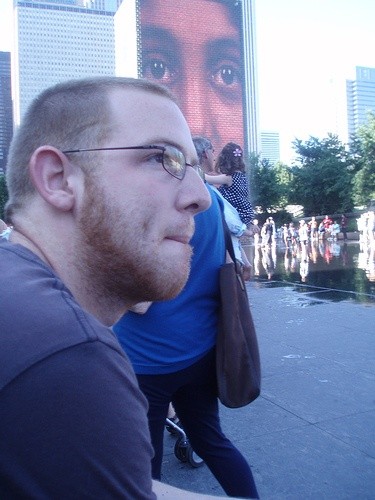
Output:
[62,145,206,185]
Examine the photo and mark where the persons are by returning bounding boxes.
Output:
[193,136,259,276]
[253,214,349,248]
[0,200,15,235]
[112,179,261,500]
[139,0,247,188]
[204,143,255,282]
[0,77,212,499]
[356,211,375,242]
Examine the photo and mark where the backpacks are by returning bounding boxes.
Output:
[213,189,262,409]
